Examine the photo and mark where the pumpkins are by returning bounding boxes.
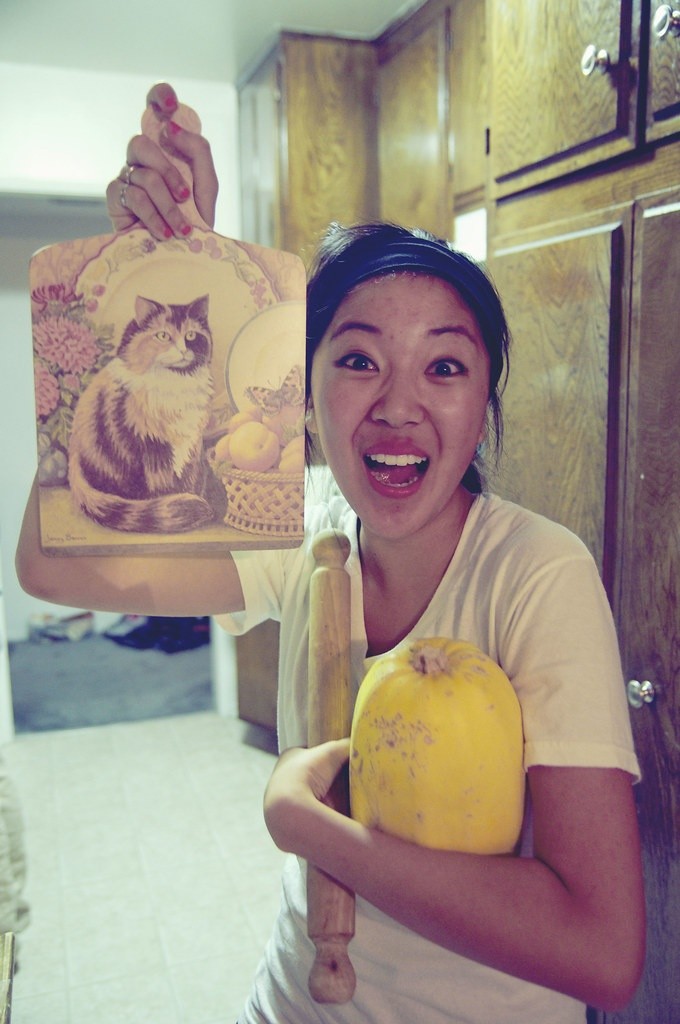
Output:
[350,638,526,857]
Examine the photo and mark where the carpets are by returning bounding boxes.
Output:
[8,637,213,737]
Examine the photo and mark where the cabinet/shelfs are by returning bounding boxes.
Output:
[484,0,680,208]
[230,26,376,274]
[474,186,680,1024]
[369,0,485,242]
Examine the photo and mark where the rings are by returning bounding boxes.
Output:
[121,185,132,207]
[127,165,140,185]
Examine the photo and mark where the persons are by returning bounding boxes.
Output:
[16,84,644,1024]
